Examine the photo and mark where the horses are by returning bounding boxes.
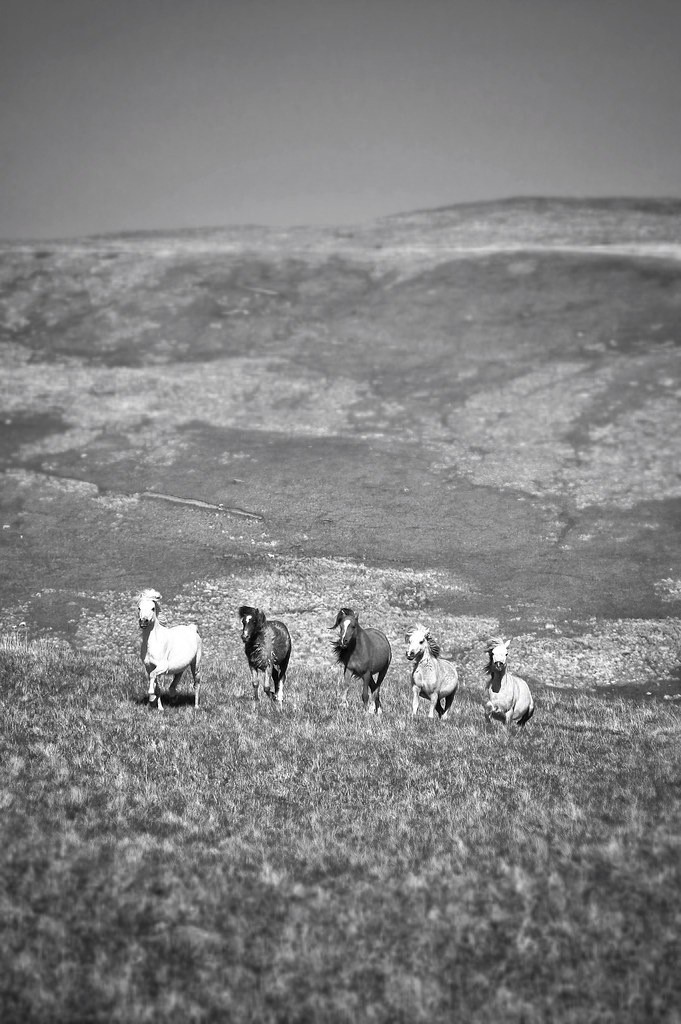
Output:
[136,588,203,712]
[327,608,392,715]
[481,636,535,727]
[238,605,292,709]
[404,622,460,721]
